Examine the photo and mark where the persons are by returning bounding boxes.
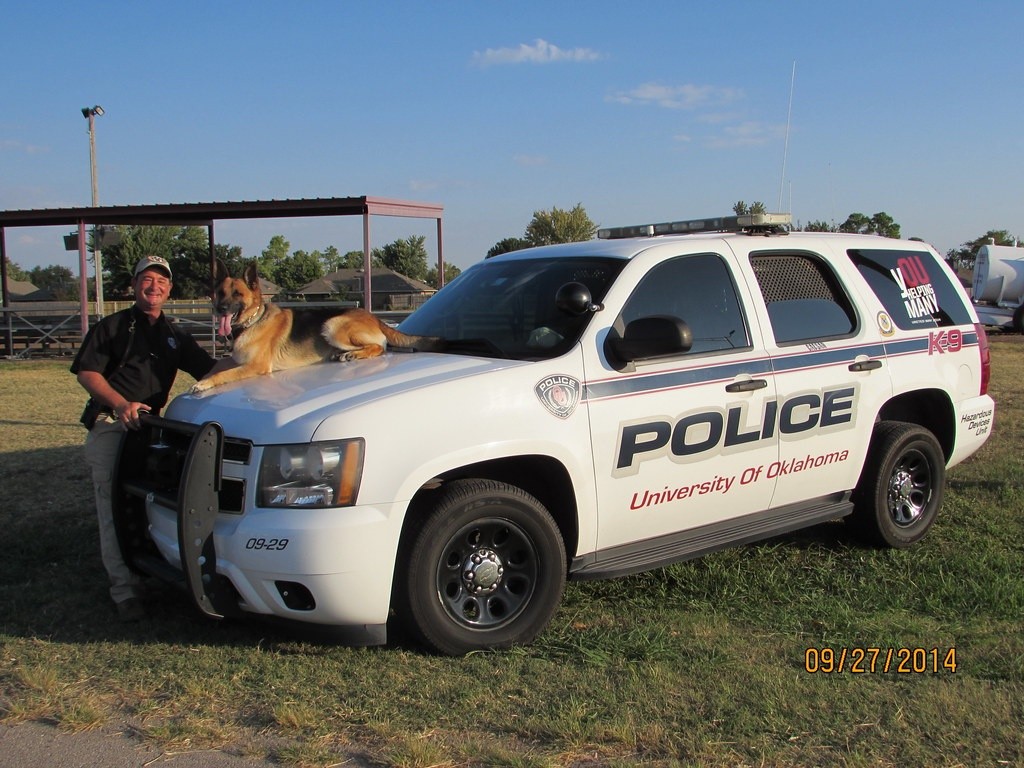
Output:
[77,256,224,621]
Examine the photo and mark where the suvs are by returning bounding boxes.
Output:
[107,212,996,661]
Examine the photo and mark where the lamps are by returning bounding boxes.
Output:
[63,231,79,251]
[99,229,123,247]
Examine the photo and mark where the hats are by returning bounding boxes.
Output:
[134,256,172,282]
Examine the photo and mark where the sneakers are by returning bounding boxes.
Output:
[117,598,145,623]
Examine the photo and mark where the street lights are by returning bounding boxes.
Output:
[63,105,124,323]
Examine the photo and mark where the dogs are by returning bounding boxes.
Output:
[188,255,440,395]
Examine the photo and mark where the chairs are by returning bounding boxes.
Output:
[673,276,743,353]
[539,278,605,339]
